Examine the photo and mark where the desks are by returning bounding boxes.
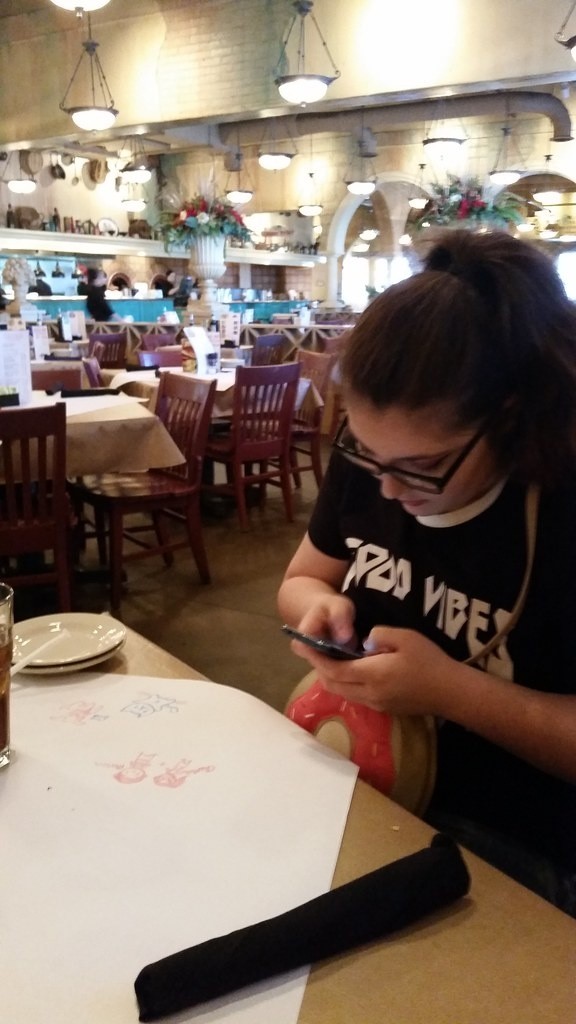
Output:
[49,336,89,349]
[155,344,255,365]
[103,365,324,507]
[86,626,576,1024]
[1,390,186,621]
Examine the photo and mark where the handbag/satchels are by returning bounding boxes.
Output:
[283,666,438,818]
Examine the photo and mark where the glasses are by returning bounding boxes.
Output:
[329,413,487,495]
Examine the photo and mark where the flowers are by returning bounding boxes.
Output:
[153,194,251,253]
[413,173,524,232]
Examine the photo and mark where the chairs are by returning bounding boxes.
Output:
[0,315,357,621]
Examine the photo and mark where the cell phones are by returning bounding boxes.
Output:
[281,624,362,659]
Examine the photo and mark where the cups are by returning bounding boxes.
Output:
[0,582,14,769]
[181,339,196,372]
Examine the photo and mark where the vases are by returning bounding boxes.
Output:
[188,233,228,279]
[408,226,457,261]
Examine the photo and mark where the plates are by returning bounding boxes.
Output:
[11,639,132,674]
[11,613,126,666]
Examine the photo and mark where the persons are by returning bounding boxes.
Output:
[163,270,179,296]
[1,258,36,314]
[84,269,113,322]
[279,229,576,918]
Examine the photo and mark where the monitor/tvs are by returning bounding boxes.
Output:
[178,276,196,296]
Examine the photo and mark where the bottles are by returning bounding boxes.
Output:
[189,313,221,374]
[6,203,99,235]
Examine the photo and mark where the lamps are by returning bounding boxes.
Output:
[50,0,111,17]
[487,120,526,184]
[115,135,152,213]
[298,173,323,217]
[269,0,342,108]
[408,162,435,210]
[553,1,576,62]
[225,127,255,203]
[343,108,378,196]
[422,101,468,159]
[0,148,37,195]
[59,11,119,136]
[257,120,299,173]
